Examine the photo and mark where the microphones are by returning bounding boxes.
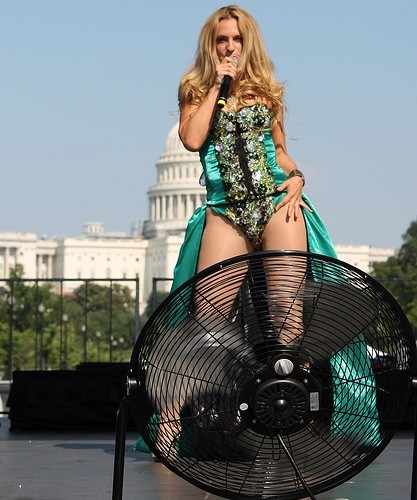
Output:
[217,57,238,107]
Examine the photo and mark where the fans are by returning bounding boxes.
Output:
[109,250,416,500]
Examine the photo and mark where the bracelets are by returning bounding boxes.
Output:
[214,77,223,85]
[288,168,305,186]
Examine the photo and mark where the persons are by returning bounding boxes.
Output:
[148,4,314,465]
